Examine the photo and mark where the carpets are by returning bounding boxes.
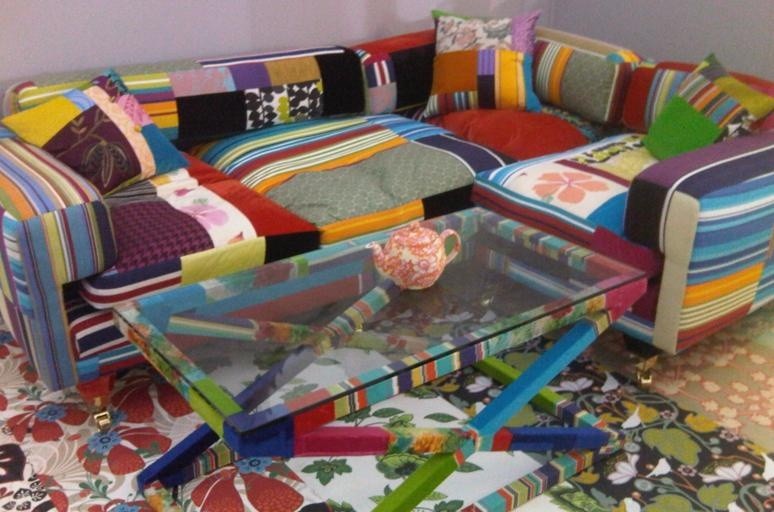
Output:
[0,281,772,512]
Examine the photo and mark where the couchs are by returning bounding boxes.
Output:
[1,28,773,393]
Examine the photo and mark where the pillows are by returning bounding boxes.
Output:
[2,67,194,200]
[418,7,544,123]
[643,50,772,162]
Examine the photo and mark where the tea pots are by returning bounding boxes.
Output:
[366,220,462,293]
